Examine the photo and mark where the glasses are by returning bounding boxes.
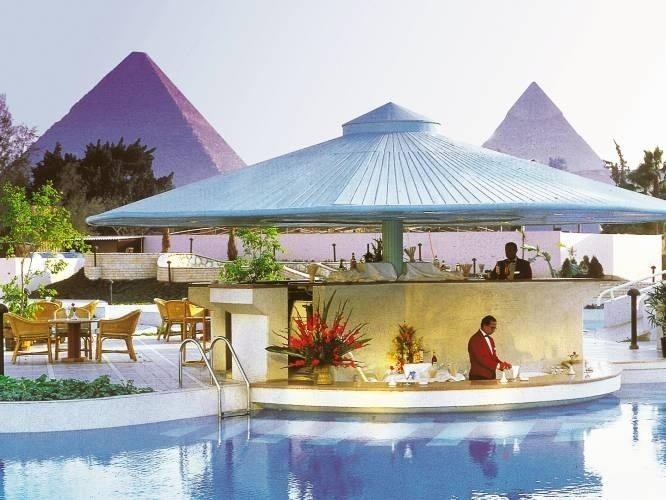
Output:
[488,326,497,332]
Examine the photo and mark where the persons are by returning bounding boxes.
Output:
[492,241,532,279]
[468,314,511,380]
[468,424,499,478]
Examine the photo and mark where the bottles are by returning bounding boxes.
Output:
[432,352,437,366]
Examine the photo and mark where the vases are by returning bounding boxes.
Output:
[314,366,333,385]
[662,338,665,357]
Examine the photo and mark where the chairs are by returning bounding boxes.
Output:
[6,297,195,364]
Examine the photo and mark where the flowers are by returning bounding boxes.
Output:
[644,281,666,336]
[387,319,430,374]
[263,290,372,371]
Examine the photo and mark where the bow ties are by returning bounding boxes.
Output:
[506,260,516,264]
